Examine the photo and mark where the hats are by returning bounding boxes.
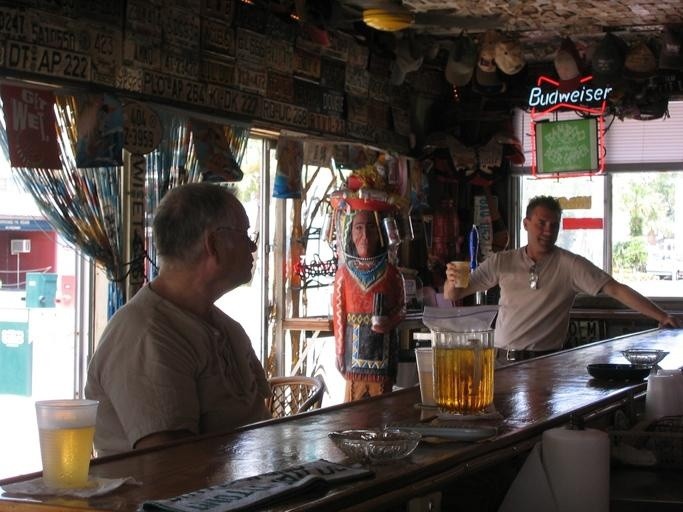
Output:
[304,1,336,47]
[659,24,682,69]
[371,32,439,98]
[445,28,475,86]
[477,29,506,85]
[554,35,584,90]
[591,33,630,83]
[408,117,524,187]
[494,31,527,88]
[626,41,656,79]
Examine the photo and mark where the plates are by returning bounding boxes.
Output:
[587,363,653,380]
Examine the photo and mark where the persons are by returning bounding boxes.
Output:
[443,194,682,371]
[333,209,405,405]
[80,181,274,463]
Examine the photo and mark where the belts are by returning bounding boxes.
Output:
[494,347,555,361]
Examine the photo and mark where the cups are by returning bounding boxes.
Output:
[415,328,498,417]
[33,396,100,491]
[452,260,472,289]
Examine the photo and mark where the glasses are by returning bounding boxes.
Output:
[529,264,539,290]
[215,227,260,246]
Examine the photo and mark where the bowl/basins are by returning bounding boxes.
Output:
[324,427,423,464]
[617,347,672,367]
